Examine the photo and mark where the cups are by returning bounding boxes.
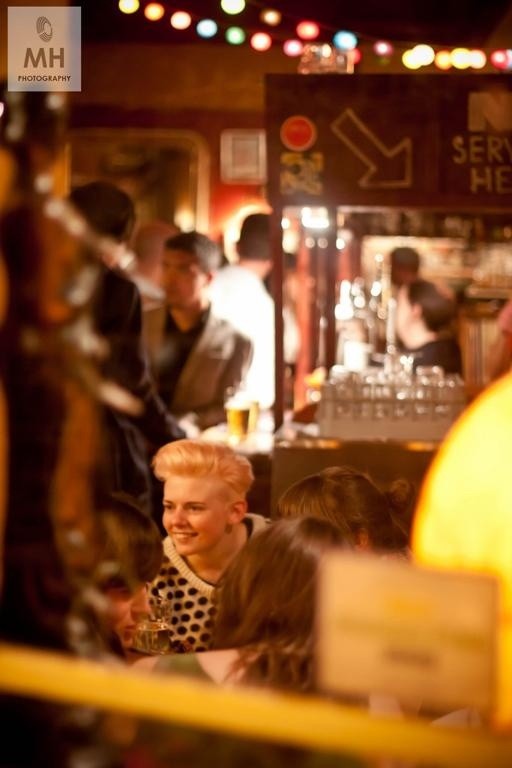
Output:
[223,387,259,439]
[133,596,173,655]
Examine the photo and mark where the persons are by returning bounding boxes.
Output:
[394,279,462,377]
[70,441,414,696]
[68,181,297,515]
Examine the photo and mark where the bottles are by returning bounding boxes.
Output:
[320,364,466,421]
